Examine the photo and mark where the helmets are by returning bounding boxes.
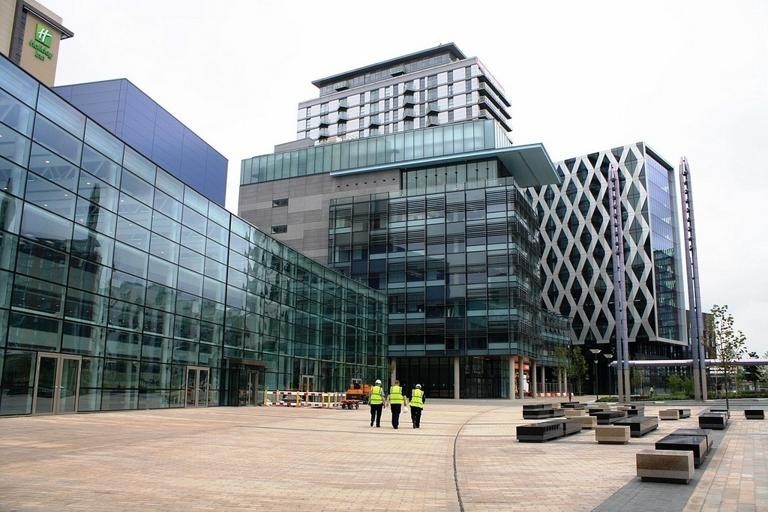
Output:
[375,379,381,384]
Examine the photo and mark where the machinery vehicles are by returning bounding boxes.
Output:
[345,378,373,404]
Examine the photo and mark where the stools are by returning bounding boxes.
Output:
[516,402,729,485]
[744,406,764,419]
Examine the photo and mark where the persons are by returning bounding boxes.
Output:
[368,379,386,428]
[649,385,655,398]
[407,383,426,429]
[385,380,407,430]
